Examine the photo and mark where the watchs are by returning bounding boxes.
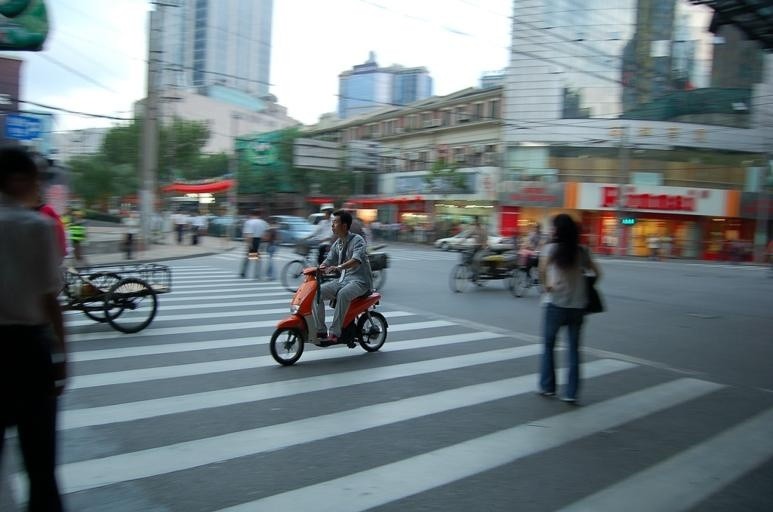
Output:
[336,264,342,270]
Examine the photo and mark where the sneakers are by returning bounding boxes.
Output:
[316,332,340,345]
[536,389,578,405]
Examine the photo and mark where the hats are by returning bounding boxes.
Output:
[0,145,56,181]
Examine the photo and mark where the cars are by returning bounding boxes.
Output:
[434,230,505,252]
[264,215,316,245]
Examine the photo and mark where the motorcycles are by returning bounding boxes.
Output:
[270,266,389,365]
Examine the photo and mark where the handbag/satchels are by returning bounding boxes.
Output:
[583,274,604,315]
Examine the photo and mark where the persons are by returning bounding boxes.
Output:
[451,216,487,282]
[58,203,281,283]
[298,208,334,266]
[23,189,68,269]
[0,144,68,511]
[350,216,488,256]
[600,230,772,266]
[532,212,601,402]
[289,208,374,345]
[515,222,545,286]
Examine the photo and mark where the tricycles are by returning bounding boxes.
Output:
[281,237,388,293]
[448,245,543,297]
[58,263,172,334]
